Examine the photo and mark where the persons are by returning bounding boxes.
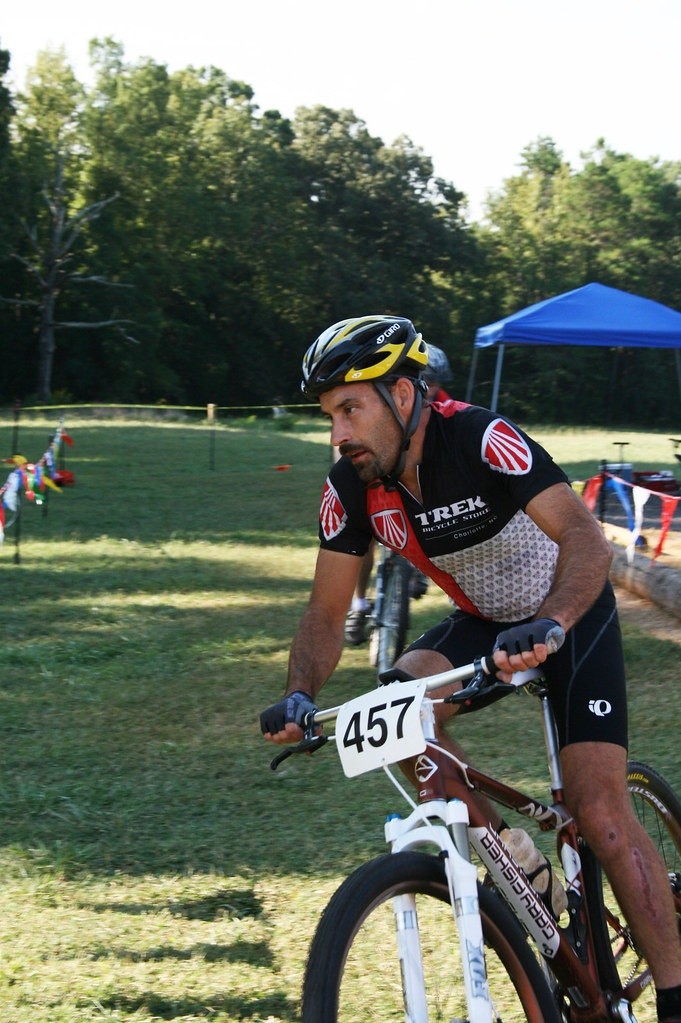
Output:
[342,342,457,645]
[259,315,681,1022]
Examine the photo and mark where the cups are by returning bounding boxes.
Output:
[571,481,584,495]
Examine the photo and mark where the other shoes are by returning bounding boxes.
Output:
[409,569,429,599]
[343,602,372,645]
[479,873,528,948]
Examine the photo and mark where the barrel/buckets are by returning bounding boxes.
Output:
[598,463,632,492]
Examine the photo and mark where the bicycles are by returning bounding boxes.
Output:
[346,554,428,680]
[269,641,678,1023]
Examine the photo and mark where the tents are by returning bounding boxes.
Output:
[468,281,681,415]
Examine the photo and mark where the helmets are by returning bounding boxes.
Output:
[301,315,429,393]
[426,343,453,384]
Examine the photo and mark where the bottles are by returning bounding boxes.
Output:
[499,828,568,919]
